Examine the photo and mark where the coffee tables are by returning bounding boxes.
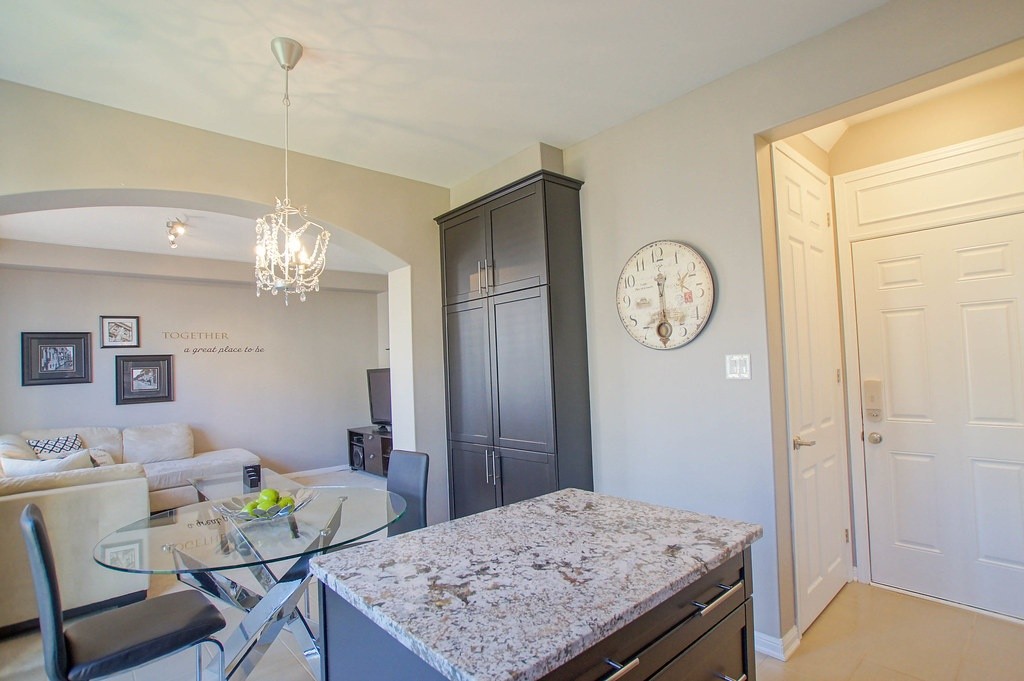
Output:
[187,468,304,499]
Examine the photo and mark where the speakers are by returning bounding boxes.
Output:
[352,445,365,471]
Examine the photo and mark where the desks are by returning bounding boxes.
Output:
[93,487,408,680]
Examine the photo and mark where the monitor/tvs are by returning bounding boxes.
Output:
[367,368,391,424]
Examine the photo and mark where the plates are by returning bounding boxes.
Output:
[211,488,321,524]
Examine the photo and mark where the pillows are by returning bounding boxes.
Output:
[0,449,94,476]
[26,433,84,459]
[38,447,115,467]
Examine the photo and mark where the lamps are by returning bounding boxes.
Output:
[167,216,186,246]
[252,36,332,301]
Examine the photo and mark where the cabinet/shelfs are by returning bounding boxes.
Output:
[434,170,593,519]
[347,426,394,477]
[308,487,763,681]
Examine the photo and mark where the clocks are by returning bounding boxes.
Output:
[614,240,716,353]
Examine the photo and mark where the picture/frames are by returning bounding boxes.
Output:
[20,331,94,386]
[99,316,140,348]
[114,355,175,405]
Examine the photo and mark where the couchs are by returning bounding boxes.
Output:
[0,424,264,640]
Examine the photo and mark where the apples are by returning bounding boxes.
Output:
[242,489,296,518]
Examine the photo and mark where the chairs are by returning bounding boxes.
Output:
[386,450,426,538]
[19,503,226,681]
